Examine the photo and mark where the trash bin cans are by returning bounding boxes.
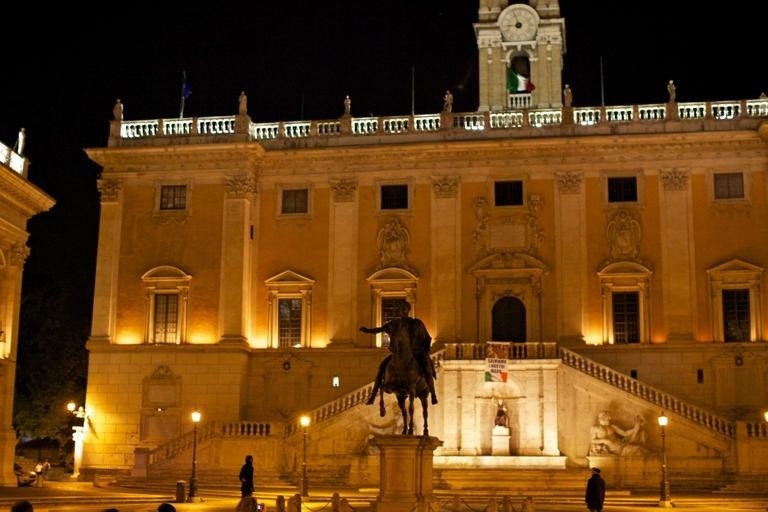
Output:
[176,481,186,502]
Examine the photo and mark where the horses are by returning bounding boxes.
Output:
[379,320,431,436]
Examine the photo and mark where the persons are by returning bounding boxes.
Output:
[759,92,768,116]
[41,458,51,489]
[238,91,248,115]
[666,79,677,104]
[343,95,351,115]
[357,301,438,405]
[10,502,32,512]
[443,90,454,113]
[33,461,42,489]
[113,98,124,121]
[157,504,176,512]
[584,466,605,512]
[563,84,574,107]
[487,384,511,429]
[238,454,255,498]
[588,408,650,456]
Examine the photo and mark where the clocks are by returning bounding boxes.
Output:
[497,4,540,41]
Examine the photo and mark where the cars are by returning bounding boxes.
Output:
[14,464,37,486]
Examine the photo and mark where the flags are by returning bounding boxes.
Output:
[504,63,536,92]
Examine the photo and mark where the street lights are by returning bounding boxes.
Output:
[67,402,76,436]
[190,412,201,496]
[658,410,670,499]
[298,415,311,495]
